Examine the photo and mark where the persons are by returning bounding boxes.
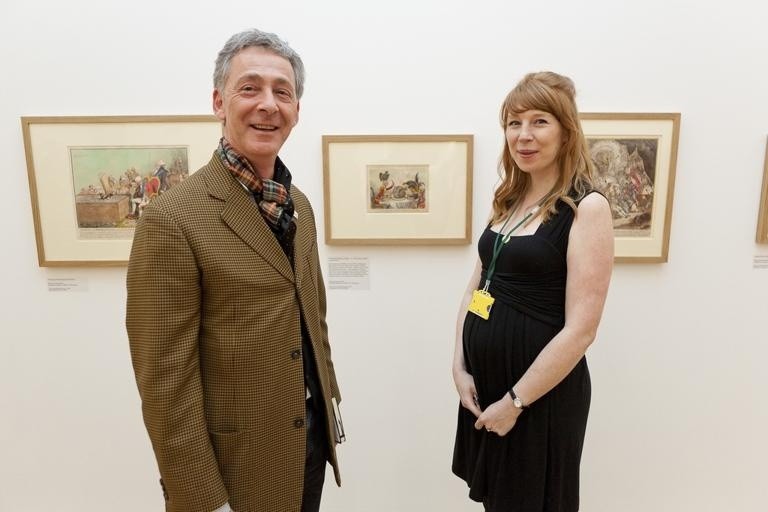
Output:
[126,28,343,511]
[451,72,615,512]
[108,161,170,219]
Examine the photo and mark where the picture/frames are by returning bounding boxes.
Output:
[321,134,473,246]
[21,116,225,268]
[578,113,682,263]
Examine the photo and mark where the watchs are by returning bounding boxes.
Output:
[507,387,529,410]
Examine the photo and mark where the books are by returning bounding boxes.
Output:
[331,396,346,443]
